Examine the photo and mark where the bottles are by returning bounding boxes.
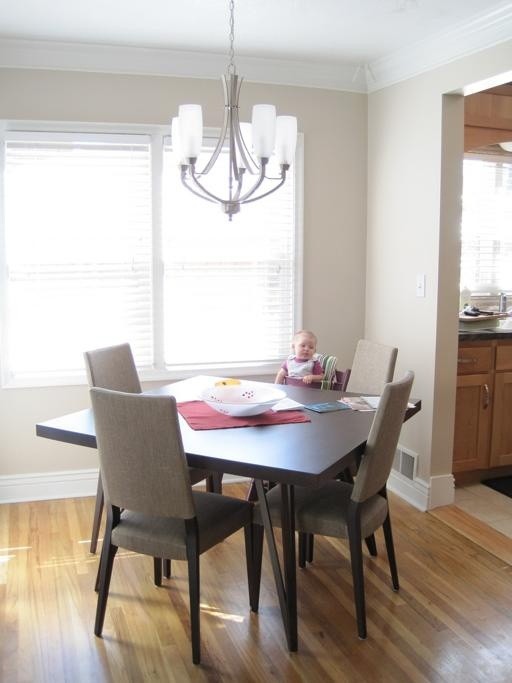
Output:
[499,292,506,312]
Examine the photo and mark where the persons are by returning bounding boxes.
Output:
[275,329,324,385]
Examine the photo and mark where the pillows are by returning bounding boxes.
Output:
[311,351,337,388]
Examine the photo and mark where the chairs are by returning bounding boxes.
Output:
[306,338,399,562]
[89,385,257,663]
[83,342,223,578]
[284,369,350,392]
[251,370,415,639]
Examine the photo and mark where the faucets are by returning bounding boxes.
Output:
[499,292,512,314]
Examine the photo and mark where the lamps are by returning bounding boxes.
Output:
[171,1,299,224]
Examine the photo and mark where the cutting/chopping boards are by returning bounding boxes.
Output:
[459,306,512,322]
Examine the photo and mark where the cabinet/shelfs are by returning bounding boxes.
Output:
[452,338,512,485]
